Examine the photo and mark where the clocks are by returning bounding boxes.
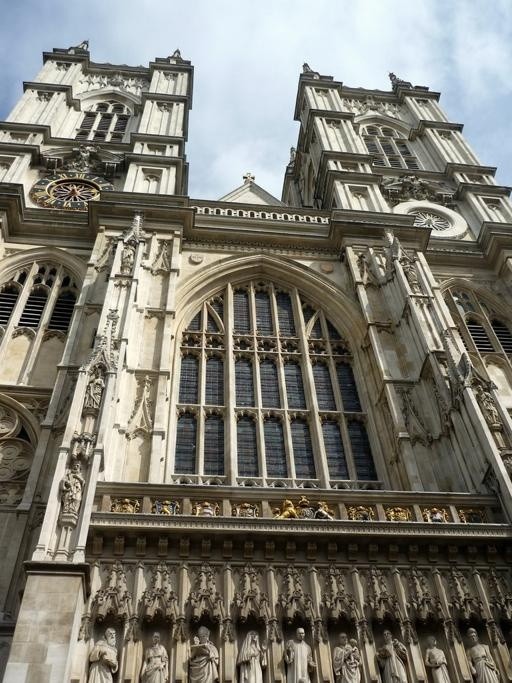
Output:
[29,171,114,210]
[394,201,468,239]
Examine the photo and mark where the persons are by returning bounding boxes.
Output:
[235,628,268,682]
[346,637,360,665]
[377,628,410,682]
[333,630,363,682]
[466,626,500,682]
[138,630,169,682]
[476,384,502,424]
[424,634,453,682]
[59,459,85,514]
[188,624,220,682]
[87,626,120,682]
[284,627,317,682]
